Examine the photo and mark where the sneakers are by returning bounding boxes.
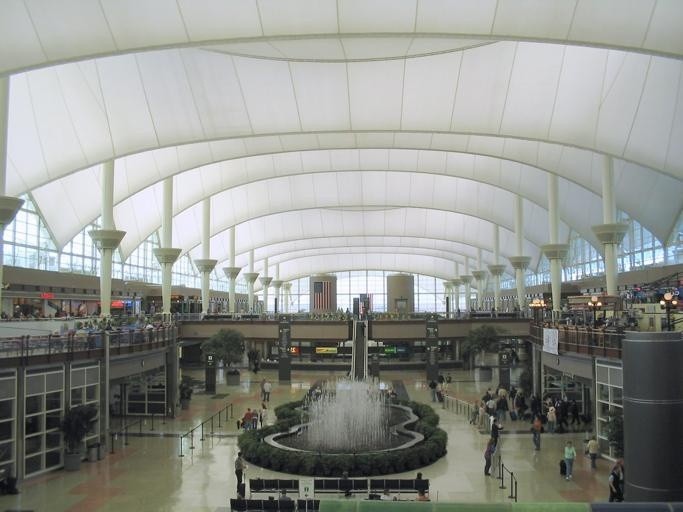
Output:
[565,475,572,480]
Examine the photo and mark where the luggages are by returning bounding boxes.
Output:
[560,460,566,475]
[239,473,245,497]
[510,411,517,421]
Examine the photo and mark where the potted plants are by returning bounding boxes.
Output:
[59,405,98,471]
[180,384,192,411]
[226,370,240,385]
[474,366,492,381]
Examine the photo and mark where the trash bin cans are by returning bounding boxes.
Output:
[227,371,240,385]
[479,367,492,382]
[87,442,105,462]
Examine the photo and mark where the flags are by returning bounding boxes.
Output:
[359,293,373,316]
[313,280,331,311]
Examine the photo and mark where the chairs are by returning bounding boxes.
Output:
[230,478,428,512]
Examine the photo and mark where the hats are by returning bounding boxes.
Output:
[549,406,556,413]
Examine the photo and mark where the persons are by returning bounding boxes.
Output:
[259,377,265,398]
[234,452,245,492]
[343,471,351,497]
[483,438,495,474]
[381,488,399,500]
[263,380,272,401]
[415,471,428,499]
[476,402,485,428]
[469,401,479,425]
[481,382,581,433]
[564,441,576,480]
[268,495,274,499]
[491,418,502,445]
[241,407,263,431]
[613,457,623,494]
[532,414,542,450]
[278,490,290,500]
[586,435,599,467]
[608,464,622,502]
[428,372,451,401]
